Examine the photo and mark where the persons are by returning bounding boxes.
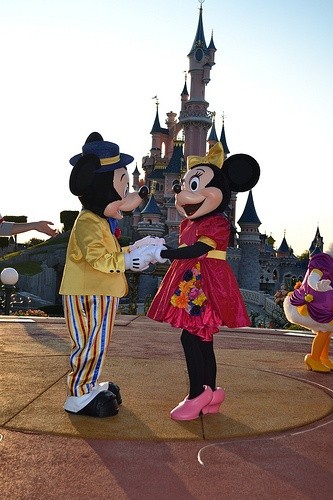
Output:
[0,215,59,237]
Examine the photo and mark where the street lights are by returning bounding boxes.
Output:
[0,267,19,315]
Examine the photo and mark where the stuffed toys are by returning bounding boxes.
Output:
[282,236,333,372]
[58,131,149,418]
[143,141,261,420]
